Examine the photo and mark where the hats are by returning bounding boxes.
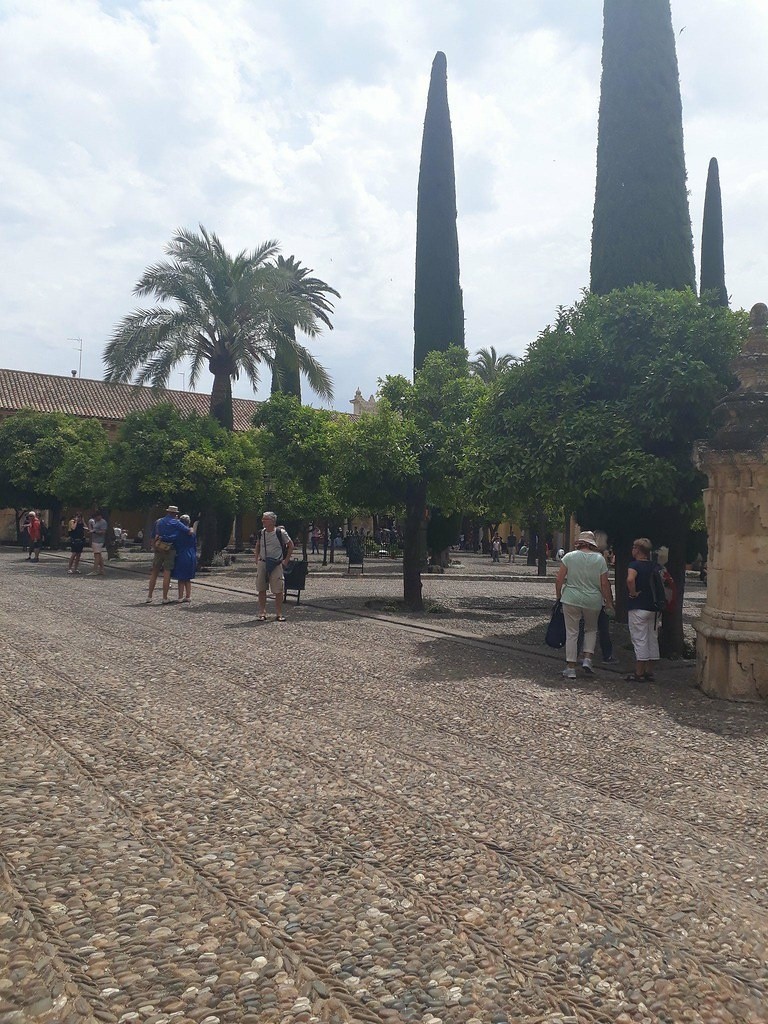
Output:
[165,506,179,513]
[94,510,101,517]
[579,531,598,548]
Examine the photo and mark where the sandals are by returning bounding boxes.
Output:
[624,671,653,682]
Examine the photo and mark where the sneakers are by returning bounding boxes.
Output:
[582,658,594,673]
[563,665,576,679]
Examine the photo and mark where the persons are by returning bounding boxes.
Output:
[114,524,122,546]
[491,532,503,563]
[624,539,678,684]
[507,531,517,563]
[555,531,615,679]
[480,536,485,554]
[568,529,620,665]
[146,505,199,603]
[120,530,127,548]
[21,511,47,564]
[64,509,108,576]
[518,536,538,556]
[308,526,396,555]
[254,512,294,622]
[699,561,707,586]
[558,547,564,559]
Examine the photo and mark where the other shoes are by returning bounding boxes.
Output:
[162,597,174,604]
[174,597,190,603]
[75,569,82,574]
[146,597,152,602]
[67,569,73,574]
[85,571,97,576]
[97,571,104,575]
[602,655,620,664]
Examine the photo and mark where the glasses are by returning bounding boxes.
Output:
[261,518,273,522]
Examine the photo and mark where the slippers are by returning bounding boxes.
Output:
[277,615,286,621]
[258,616,267,621]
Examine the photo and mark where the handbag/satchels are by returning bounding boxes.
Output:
[156,540,173,552]
[265,556,281,571]
[545,599,566,648]
[74,539,82,547]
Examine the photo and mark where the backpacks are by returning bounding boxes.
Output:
[651,561,675,613]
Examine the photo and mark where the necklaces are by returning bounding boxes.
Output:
[639,557,648,559]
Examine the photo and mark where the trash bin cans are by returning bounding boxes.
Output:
[349,535,364,564]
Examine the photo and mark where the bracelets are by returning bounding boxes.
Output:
[629,594,636,597]
